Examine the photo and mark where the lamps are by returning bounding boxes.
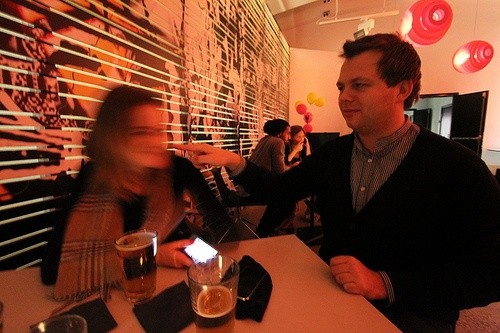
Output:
[399,0,495,75]
[353,17,375,39]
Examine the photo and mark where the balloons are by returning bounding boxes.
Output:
[304,124,312,132]
[307,92,325,107]
[303,112,314,123]
[295,100,303,110]
[296,104,306,115]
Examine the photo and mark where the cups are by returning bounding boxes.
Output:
[113,228,159,305]
[38,315,87,333]
[187,254,240,333]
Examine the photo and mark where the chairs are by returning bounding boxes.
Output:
[210,167,270,244]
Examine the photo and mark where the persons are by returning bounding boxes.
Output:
[249,118,300,237]
[285,125,312,166]
[173,33,500,333]
[39,87,240,301]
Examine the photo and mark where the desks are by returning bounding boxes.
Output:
[281,161,325,247]
[0,234,402,333]
